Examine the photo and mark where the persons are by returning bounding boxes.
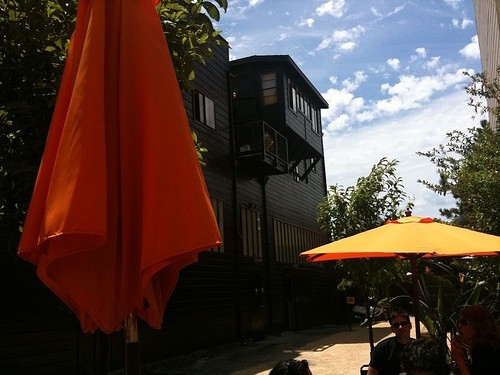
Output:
[366,305,500,375]
[268,358,312,375]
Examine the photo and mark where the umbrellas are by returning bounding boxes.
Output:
[17,0,224,375]
[299,208,500,339]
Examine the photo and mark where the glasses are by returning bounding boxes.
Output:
[460,318,471,326]
[288,358,296,375]
[392,320,409,329]
